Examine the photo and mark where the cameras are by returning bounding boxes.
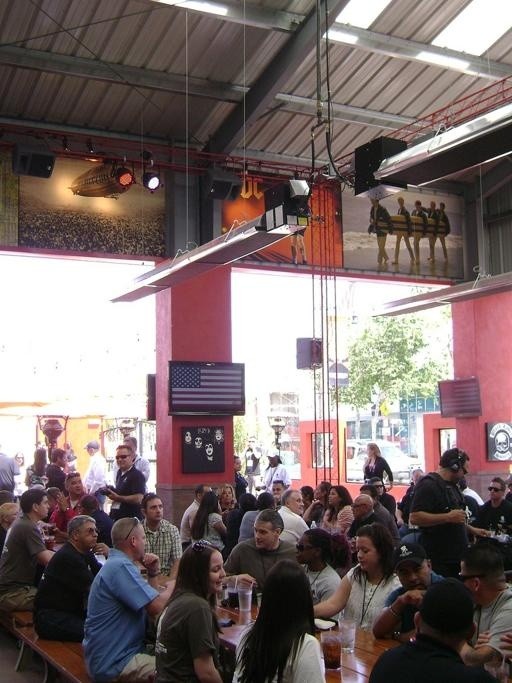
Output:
[100,485,114,495]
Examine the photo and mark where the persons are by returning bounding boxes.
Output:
[368,197,451,266]
[291,204,309,265]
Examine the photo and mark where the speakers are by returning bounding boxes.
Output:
[200,168,242,201]
[297,337,323,370]
[12,142,56,178]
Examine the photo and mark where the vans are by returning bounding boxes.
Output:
[343,439,423,484]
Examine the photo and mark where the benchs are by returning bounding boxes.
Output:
[0,604,93,680]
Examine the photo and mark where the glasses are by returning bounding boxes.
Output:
[295,543,315,551]
[487,486,503,492]
[192,538,212,552]
[115,454,132,459]
[458,572,486,582]
[350,484,384,509]
[125,516,140,540]
[76,527,101,534]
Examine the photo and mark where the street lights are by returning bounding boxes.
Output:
[36,412,71,464]
[115,414,140,440]
[266,415,289,455]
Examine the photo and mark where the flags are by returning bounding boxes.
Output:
[171,365,242,410]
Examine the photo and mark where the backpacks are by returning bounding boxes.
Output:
[395,474,455,531]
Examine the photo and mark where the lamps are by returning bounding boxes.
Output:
[352,135,407,201]
[373,0,512,201]
[106,1,293,306]
[363,160,512,318]
[253,179,314,238]
[108,147,164,195]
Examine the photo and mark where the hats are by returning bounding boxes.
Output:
[84,441,100,451]
[417,578,477,634]
[392,541,429,571]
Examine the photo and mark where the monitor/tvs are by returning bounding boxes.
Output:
[437,376,483,418]
[167,360,245,417]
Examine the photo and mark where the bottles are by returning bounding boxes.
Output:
[216,579,237,608]
[311,521,317,528]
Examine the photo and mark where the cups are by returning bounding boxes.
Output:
[320,629,342,670]
[488,520,512,540]
[40,520,55,550]
[483,660,511,682]
[238,610,252,625]
[237,583,253,611]
[321,523,342,535]
[337,617,356,654]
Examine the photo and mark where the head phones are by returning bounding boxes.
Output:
[447,448,465,473]
